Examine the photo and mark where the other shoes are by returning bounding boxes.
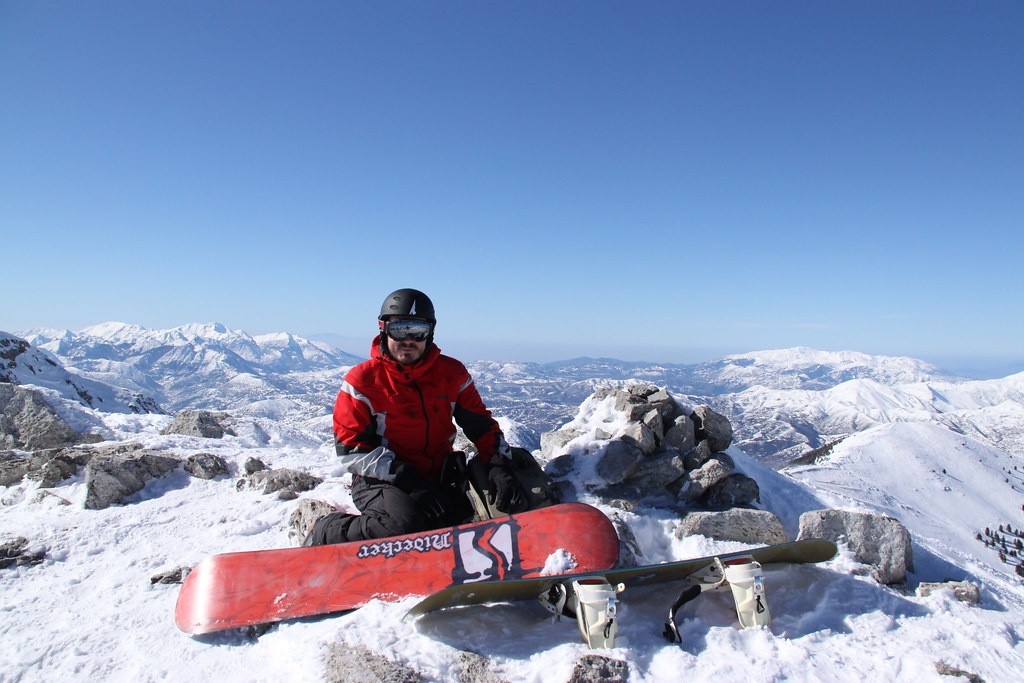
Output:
[303,515,325,547]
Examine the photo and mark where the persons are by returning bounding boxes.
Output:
[300,288,524,548]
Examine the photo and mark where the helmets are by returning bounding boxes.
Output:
[378,288,436,321]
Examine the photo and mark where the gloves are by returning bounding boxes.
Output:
[488,454,530,514]
[389,461,420,494]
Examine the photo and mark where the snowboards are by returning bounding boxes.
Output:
[174,501,621,637]
[401,536,838,624]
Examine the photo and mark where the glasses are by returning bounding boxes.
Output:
[379,320,433,342]
